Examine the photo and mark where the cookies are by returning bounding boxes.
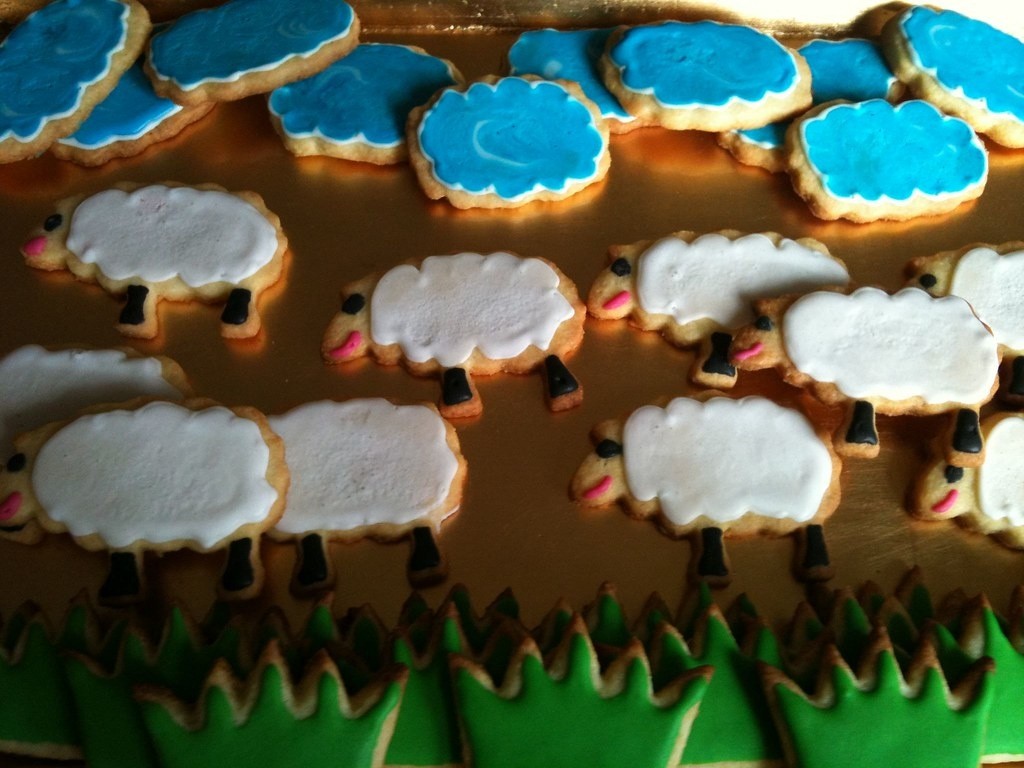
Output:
[0,0,1024,768]
